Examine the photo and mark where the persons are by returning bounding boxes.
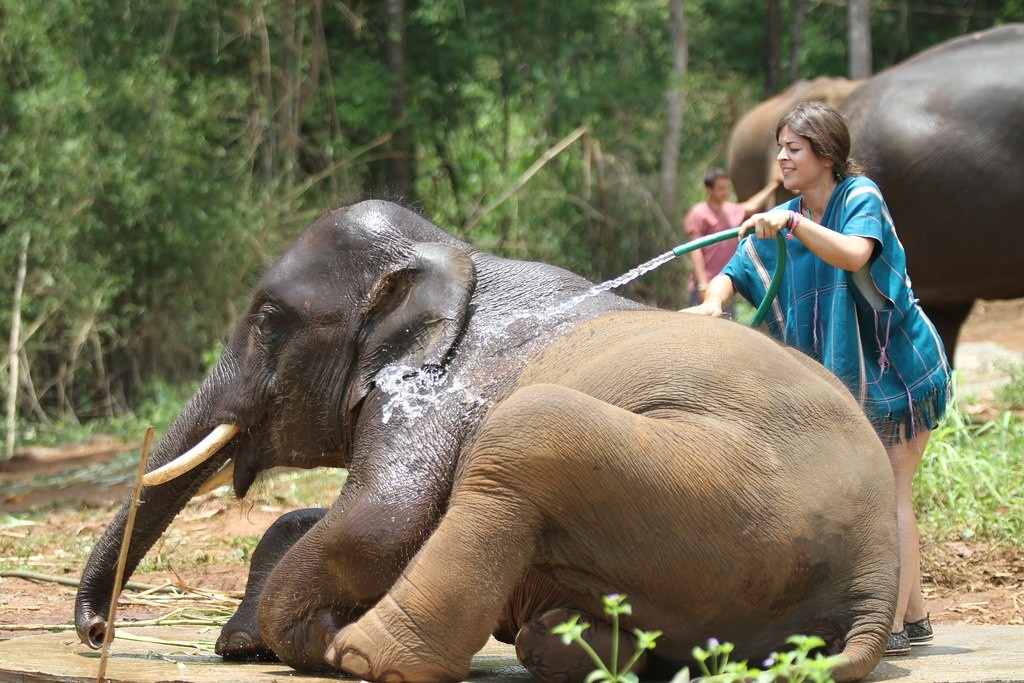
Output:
[683,170,784,308]
[680,101,953,655]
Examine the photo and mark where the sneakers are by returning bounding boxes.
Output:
[904,617,934,643]
[883,629,911,656]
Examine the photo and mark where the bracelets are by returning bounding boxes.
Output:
[786,210,803,240]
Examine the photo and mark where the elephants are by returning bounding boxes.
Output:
[76,199,899,683]
[724,24,1024,369]
